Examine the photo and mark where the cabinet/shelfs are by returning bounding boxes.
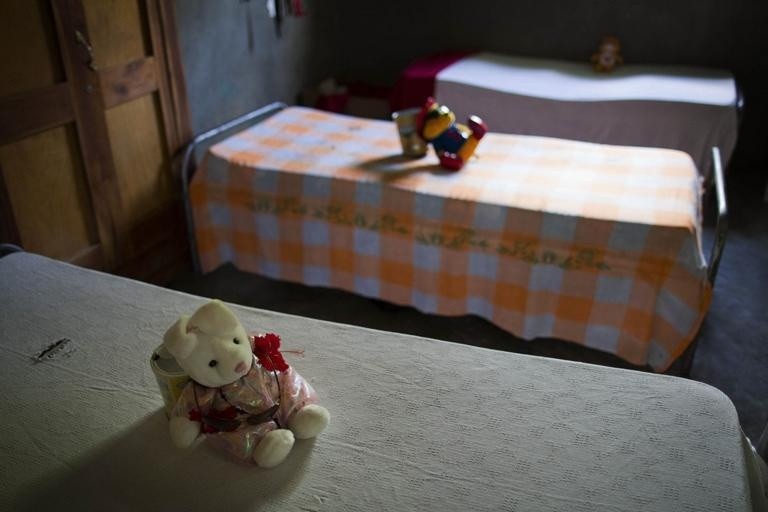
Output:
[0,0,193,270]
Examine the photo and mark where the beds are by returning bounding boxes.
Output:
[182,100,730,373]
[386,50,744,188]
[0,243,768,512]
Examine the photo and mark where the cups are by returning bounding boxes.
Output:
[150,342,189,419]
[393,108,431,158]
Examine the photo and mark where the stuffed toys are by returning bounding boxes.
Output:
[161,298,332,467]
